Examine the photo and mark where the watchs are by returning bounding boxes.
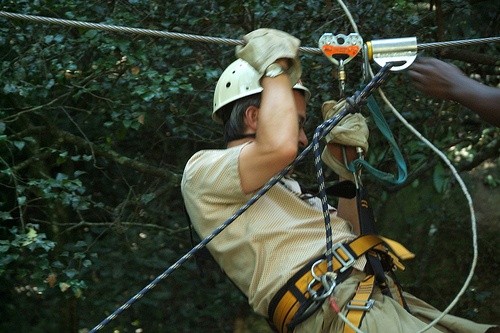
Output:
[261,63,287,78]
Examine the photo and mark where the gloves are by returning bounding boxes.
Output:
[320,97,368,181]
[234,28,304,90]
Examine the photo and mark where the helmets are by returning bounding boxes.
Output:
[211,58,311,125]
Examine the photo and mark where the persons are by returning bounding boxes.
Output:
[402,55,500,116]
[172,31,500,333]
